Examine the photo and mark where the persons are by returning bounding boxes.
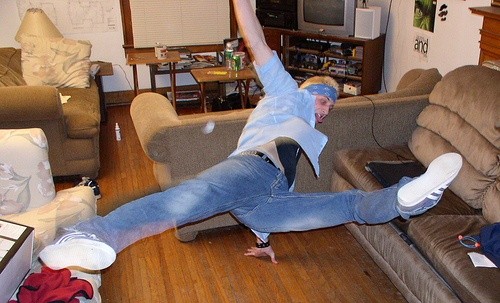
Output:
[39,0,463,272]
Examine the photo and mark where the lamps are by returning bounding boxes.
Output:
[15,8,64,42]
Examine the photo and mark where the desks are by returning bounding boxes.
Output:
[149,58,226,101]
[126,51,180,108]
[190,65,256,113]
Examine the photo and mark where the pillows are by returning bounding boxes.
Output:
[20,35,92,88]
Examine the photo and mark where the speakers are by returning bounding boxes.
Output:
[354,7,381,39]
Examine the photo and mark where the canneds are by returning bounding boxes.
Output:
[225,50,245,72]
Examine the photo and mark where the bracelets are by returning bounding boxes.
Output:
[255,241,271,247]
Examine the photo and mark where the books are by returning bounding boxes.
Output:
[167,89,209,102]
[156,55,220,71]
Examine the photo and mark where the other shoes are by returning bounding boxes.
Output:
[75,176,101,200]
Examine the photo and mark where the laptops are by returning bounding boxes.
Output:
[366,160,426,189]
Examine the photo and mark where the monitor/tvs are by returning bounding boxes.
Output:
[296,0,357,37]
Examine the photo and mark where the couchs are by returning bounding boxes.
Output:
[330,65,500,303]
[0,47,102,179]
[130,68,442,244]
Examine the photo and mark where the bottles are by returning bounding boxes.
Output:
[236,52,245,69]
[225,43,234,71]
[115,123,121,141]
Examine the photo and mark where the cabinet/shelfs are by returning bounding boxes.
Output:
[263,28,386,96]
[469,5,500,67]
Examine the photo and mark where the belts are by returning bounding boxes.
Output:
[240,149,281,171]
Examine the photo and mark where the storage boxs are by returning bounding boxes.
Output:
[344,84,361,95]
[0,219,35,303]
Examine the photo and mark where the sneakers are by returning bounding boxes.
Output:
[396,153,463,213]
[39,227,116,271]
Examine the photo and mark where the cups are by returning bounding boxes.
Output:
[154,46,163,57]
[156,48,167,60]
